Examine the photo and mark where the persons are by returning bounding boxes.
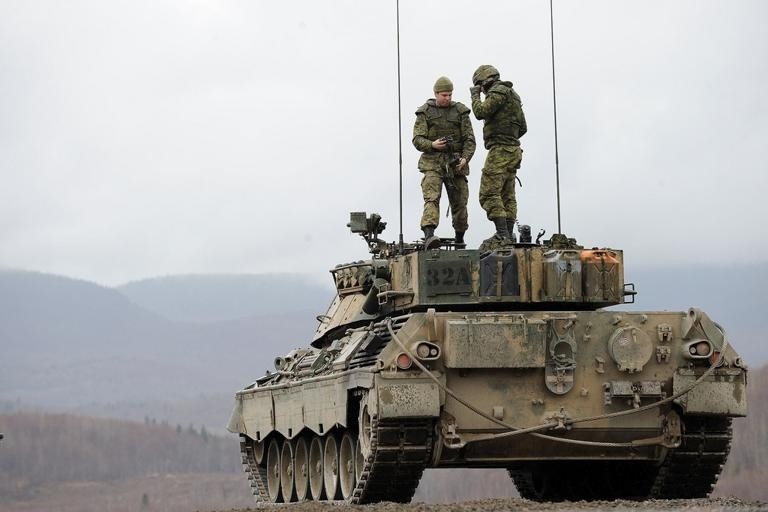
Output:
[412,76,476,250]
[470,65,527,244]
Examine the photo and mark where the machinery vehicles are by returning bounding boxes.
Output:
[226,212,748,506]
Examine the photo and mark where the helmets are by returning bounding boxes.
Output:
[473,65,500,86]
[434,76,453,92]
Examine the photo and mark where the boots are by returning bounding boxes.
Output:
[493,217,514,244]
[423,225,441,252]
[455,231,465,251]
